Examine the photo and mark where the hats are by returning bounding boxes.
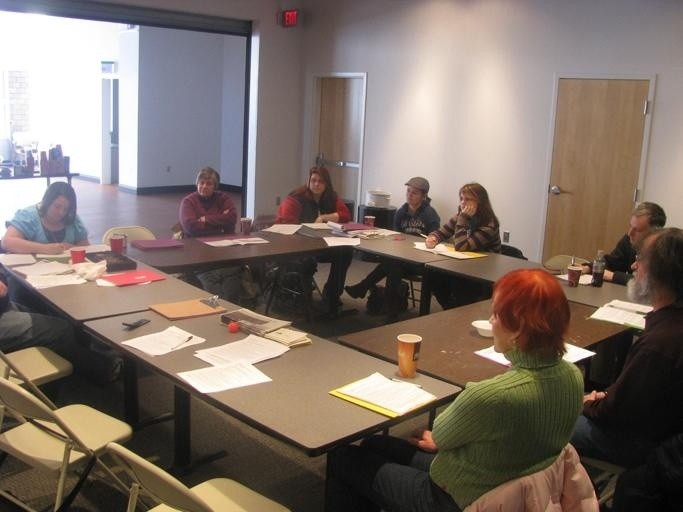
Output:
[405,177,430,192]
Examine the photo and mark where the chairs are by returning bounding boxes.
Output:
[0,137,27,168]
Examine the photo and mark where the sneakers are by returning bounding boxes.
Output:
[111,357,124,381]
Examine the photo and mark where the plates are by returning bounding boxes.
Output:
[470,319,495,338]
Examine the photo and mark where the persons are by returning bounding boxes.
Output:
[569,226,683,466]
[425,182,501,312]
[571,202,666,381]
[278,165,351,305]
[340,265,584,512]
[179,167,245,307]
[0,264,124,387]
[1,182,90,255]
[344,177,440,318]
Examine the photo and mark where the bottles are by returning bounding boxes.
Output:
[591,250,605,288]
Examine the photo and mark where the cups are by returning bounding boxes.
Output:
[240,217,252,235]
[364,215,376,228]
[567,265,583,288]
[70,232,128,266]
[396,333,423,379]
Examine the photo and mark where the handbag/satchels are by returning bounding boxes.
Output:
[367,281,410,316]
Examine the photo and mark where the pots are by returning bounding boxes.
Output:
[365,189,390,209]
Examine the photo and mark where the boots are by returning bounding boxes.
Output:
[345,278,374,299]
